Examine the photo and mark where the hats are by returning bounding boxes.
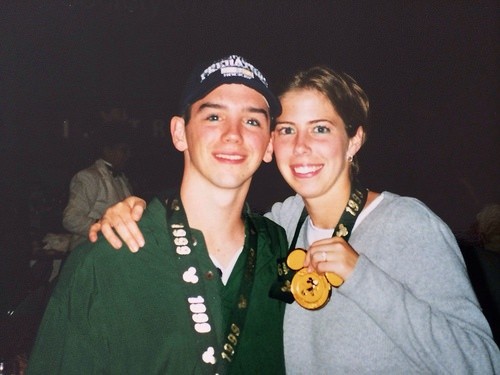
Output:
[176,52,282,117]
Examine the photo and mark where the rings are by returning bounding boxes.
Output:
[324,251,328,263]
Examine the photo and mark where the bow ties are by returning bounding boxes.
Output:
[106,164,122,178]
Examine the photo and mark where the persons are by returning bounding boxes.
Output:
[89,65,500,375]
[63,132,134,251]
[23,54,290,375]
